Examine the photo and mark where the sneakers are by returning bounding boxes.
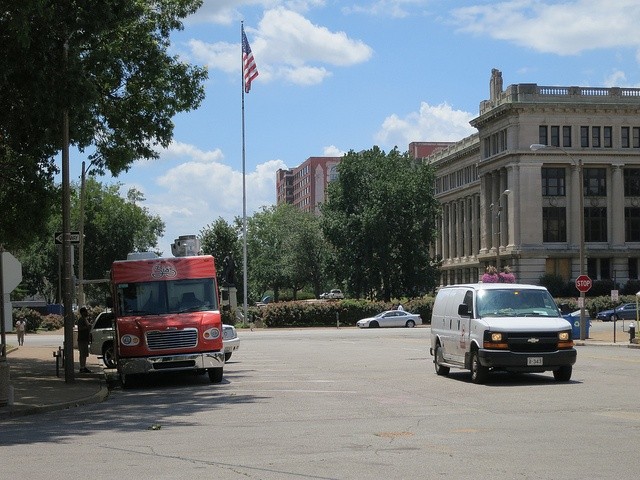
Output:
[80,367,90,373]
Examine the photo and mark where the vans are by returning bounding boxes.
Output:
[430,283,576,382]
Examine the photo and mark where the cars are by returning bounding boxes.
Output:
[320,293,332,301]
[356,309,423,328]
[596,302,640,321]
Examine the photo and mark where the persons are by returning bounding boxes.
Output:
[391,303,397,310]
[397,303,403,311]
[77,307,93,373]
[15,315,28,346]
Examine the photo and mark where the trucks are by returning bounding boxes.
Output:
[111,255,225,389]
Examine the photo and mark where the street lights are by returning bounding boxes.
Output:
[489,189,509,274]
[530,143,586,338]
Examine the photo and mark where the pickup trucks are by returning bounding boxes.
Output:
[329,289,345,298]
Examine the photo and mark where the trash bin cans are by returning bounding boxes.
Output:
[559,303,572,325]
[571,309,590,339]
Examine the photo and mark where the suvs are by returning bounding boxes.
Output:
[89,310,240,368]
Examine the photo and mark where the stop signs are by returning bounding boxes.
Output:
[575,275,592,292]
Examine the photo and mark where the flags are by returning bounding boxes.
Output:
[242,26,259,92]
